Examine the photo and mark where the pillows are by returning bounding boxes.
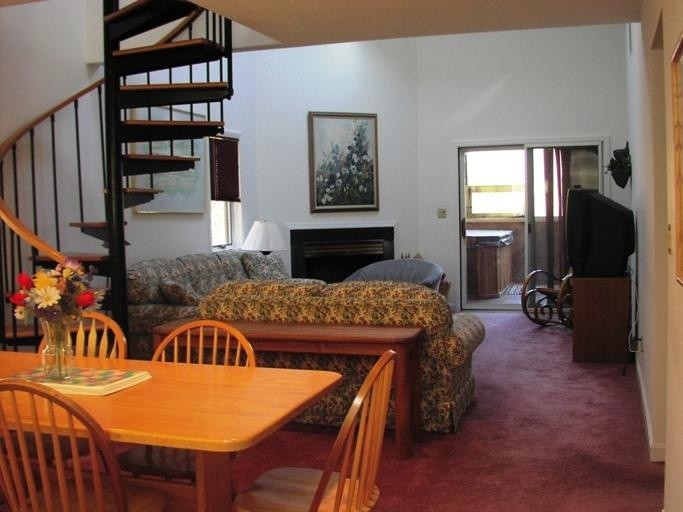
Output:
[238,250,294,285]
[158,272,205,307]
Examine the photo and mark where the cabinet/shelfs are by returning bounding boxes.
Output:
[568,275,632,364]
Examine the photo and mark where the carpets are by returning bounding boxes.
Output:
[506,281,558,296]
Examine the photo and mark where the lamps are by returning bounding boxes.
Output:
[239,219,292,255]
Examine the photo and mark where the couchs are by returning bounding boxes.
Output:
[126,249,487,439]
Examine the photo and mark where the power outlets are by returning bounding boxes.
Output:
[636,337,648,362]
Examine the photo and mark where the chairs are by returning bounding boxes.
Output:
[342,256,444,297]
[1,311,399,510]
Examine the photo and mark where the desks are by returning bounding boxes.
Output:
[150,315,427,462]
[466,229,515,299]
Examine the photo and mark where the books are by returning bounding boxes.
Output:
[0,366,151,396]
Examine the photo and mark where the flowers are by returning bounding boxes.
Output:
[7,257,109,379]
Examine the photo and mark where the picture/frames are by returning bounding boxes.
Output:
[307,110,381,215]
[129,100,208,215]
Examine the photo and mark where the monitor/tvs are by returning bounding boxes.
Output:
[565,186,635,278]
